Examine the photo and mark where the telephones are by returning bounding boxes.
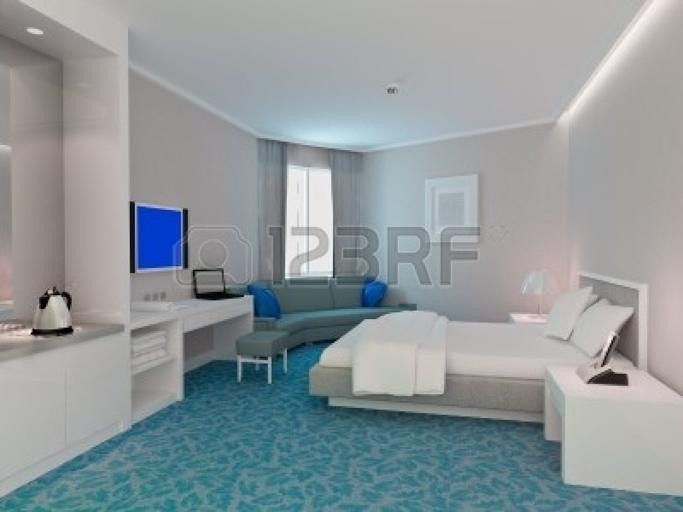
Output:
[574,330,620,385]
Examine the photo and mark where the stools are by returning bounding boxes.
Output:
[235,331,288,385]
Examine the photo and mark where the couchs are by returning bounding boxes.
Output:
[226,276,417,354]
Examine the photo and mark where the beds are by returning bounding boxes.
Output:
[309,272,649,423]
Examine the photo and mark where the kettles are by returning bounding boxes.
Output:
[32,287,73,337]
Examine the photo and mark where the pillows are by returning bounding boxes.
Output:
[247,282,280,320]
[362,277,386,307]
[544,285,598,341]
[570,298,634,358]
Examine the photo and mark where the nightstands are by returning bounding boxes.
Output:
[508,313,550,323]
[544,365,683,497]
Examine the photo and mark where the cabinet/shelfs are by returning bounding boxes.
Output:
[130,313,182,426]
[0,328,130,493]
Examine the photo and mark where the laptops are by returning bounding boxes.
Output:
[193,268,244,300]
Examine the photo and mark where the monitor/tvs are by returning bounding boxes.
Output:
[130,201,188,274]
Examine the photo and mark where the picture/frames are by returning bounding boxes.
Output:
[425,174,478,243]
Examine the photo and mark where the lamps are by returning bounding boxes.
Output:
[521,271,556,318]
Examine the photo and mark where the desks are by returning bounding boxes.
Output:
[131,294,254,373]
